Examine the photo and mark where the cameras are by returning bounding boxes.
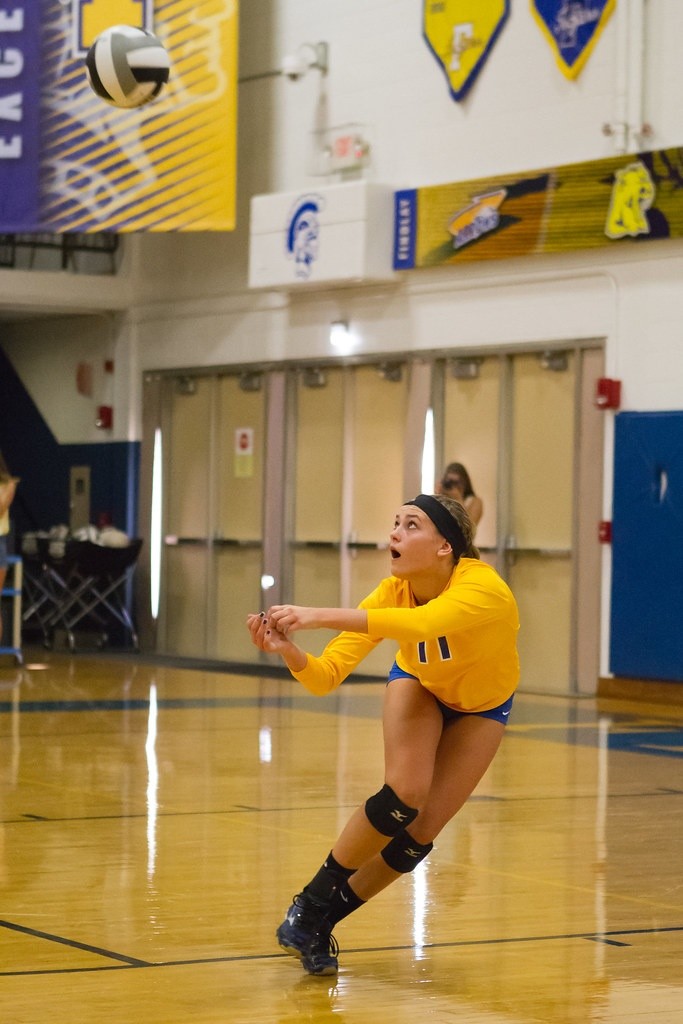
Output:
[441,478,456,489]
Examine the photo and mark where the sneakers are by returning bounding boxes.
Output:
[297,928,339,976]
[277,887,331,958]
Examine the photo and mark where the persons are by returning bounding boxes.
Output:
[245,496,522,975]
[1,470,20,593]
[437,461,483,530]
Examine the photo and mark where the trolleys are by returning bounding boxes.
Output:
[9,523,141,657]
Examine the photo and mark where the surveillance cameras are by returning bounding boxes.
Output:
[281,56,307,81]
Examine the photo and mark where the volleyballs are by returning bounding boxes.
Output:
[86,26,169,106]
